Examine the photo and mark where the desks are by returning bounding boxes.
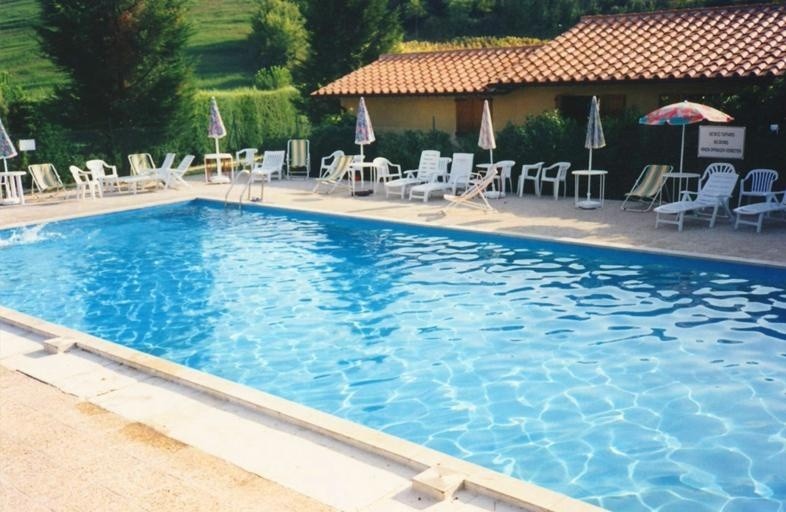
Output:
[572,170,608,209]
[1,171,26,206]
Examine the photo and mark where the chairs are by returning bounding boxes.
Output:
[68,152,195,200]
[28,163,72,205]
[315,151,571,202]
[620,165,785,231]
[204,139,310,185]
[441,166,498,216]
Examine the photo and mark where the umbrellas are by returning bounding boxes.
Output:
[584,95,606,201]
[641,97,735,204]
[355,96,376,183]
[209,97,227,177]
[1,120,18,197]
[478,99,498,191]
